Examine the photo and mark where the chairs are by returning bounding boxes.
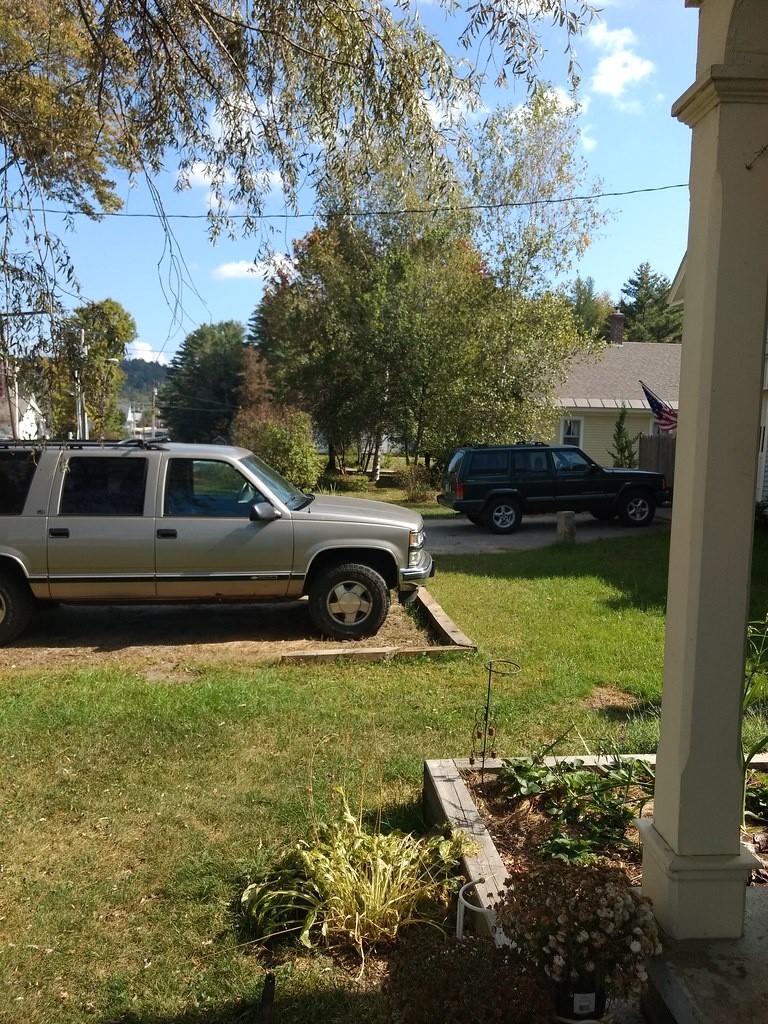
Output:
[168,463,197,511]
[533,457,544,470]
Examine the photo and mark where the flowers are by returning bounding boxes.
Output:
[486,859,663,1008]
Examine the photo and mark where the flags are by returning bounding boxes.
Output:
[642,382,677,432]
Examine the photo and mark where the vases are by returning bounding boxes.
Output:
[543,974,607,1021]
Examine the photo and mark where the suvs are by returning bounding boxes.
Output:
[0,436,433,636]
[439,444,668,533]
